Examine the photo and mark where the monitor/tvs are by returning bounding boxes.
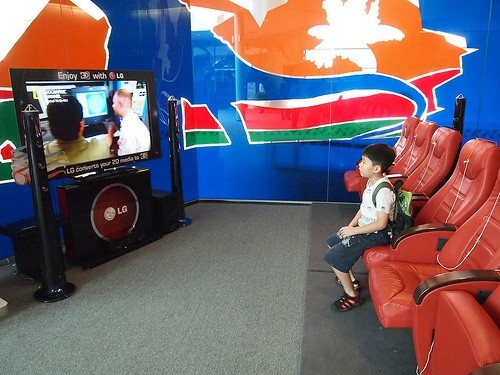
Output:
[10,68,163,182]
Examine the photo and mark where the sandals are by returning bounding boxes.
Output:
[335,276,361,292]
[333,291,362,312]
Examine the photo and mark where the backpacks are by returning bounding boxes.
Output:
[372,179,412,239]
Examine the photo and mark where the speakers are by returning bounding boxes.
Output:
[152,188,180,235]
[12,225,65,281]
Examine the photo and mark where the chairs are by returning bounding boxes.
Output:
[346,117,500,375]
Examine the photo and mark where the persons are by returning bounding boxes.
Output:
[112,89,151,156]
[42,95,115,172]
[322,143,399,312]
